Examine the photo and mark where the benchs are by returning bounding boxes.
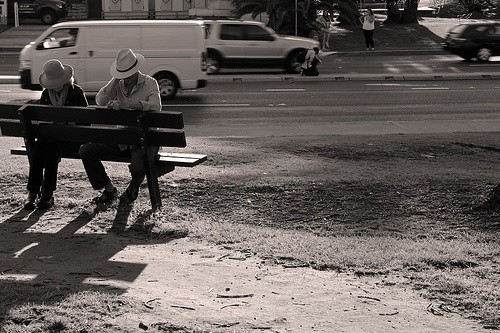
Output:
[0,103,207,209]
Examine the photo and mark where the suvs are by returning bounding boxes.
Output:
[200,17,320,73]
[444,21,500,62]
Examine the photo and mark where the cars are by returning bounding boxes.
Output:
[7,0,68,24]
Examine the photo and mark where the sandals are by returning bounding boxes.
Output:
[99,187,118,204]
[120,185,139,204]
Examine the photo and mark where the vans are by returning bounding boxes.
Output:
[19,20,208,102]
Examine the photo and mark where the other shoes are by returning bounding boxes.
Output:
[367,48,374,51]
[24,192,38,210]
[37,194,54,209]
[301,70,304,76]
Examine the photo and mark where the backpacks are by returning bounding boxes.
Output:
[296,49,312,63]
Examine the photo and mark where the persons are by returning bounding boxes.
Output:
[79,47,162,202]
[359,8,375,51]
[23,58,92,207]
[301,47,322,76]
[50,29,77,47]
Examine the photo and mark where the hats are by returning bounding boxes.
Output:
[39,59,74,89]
[110,48,144,79]
